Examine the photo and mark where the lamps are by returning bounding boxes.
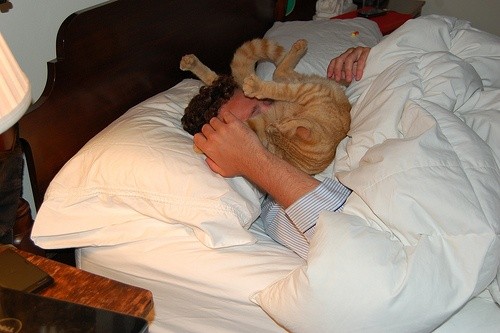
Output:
[0,32,33,243]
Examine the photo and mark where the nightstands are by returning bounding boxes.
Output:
[0,243,155,324]
[329,0,426,35]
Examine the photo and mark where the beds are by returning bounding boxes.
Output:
[17,0,500,333]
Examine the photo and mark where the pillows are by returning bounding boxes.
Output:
[30,78,266,250]
[256,17,384,81]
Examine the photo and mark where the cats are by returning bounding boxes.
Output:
[179,38,352,177]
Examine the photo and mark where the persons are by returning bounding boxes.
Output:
[181,44,372,260]
